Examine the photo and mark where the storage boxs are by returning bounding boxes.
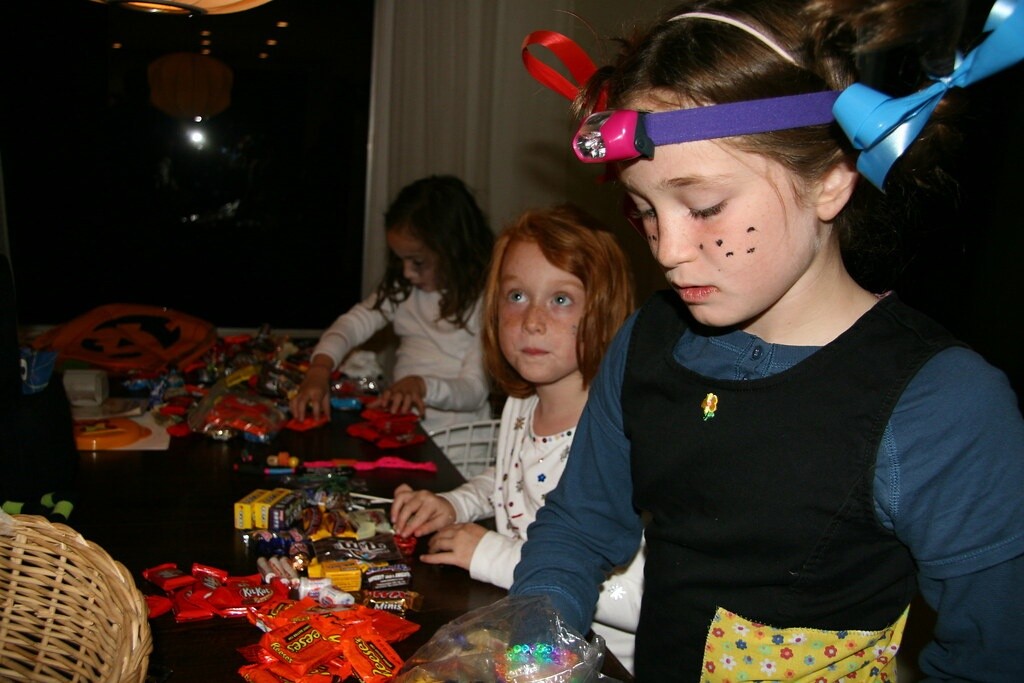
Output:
[234,488,303,529]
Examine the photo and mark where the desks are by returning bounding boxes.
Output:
[0,350,632,683]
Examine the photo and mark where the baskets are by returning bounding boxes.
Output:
[0,509,153,683]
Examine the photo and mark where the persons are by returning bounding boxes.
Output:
[506,0,1024,683]
[392,200,645,675]
[290,175,494,475]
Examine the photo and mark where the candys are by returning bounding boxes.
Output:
[137,482,421,683]
[116,324,427,449]
